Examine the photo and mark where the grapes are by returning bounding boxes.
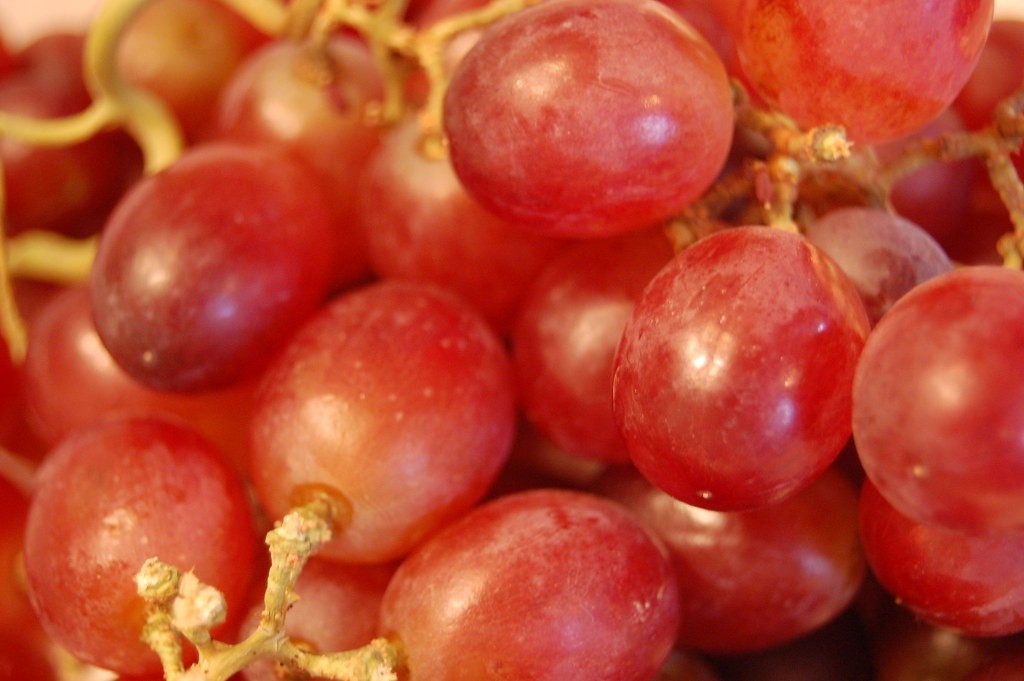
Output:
[0,0,1024,680]
